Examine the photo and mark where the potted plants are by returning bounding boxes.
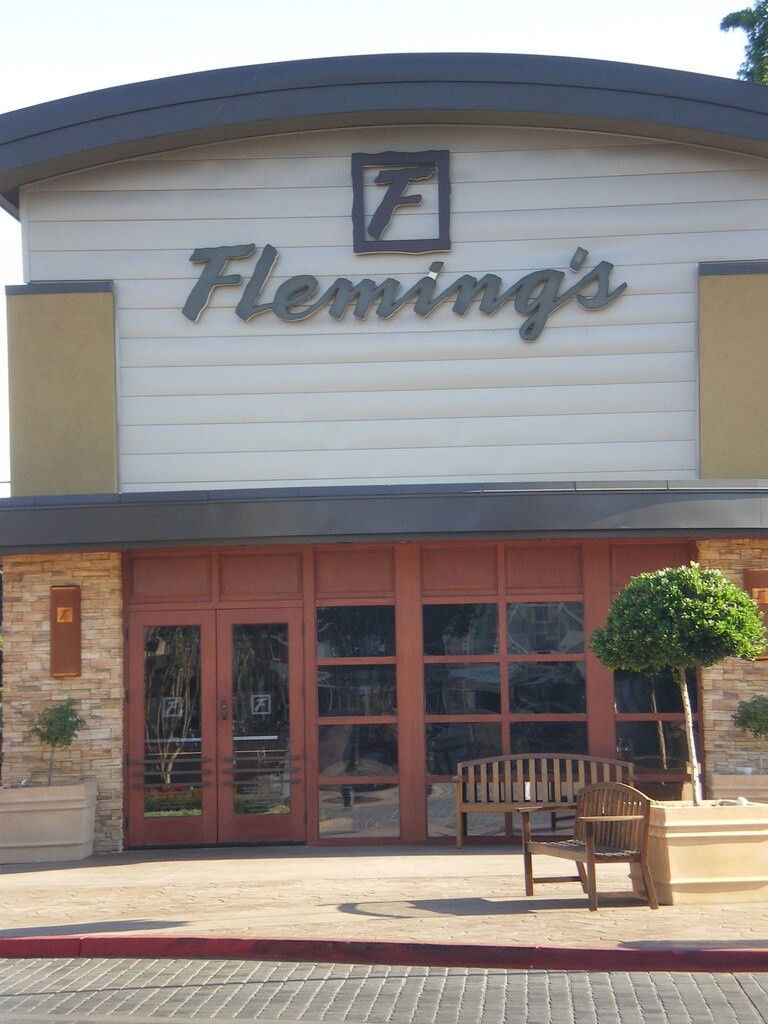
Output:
[589,560,768,907]
[0,695,99,865]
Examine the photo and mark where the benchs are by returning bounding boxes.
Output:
[513,784,658,912]
[452,753,635,848]
